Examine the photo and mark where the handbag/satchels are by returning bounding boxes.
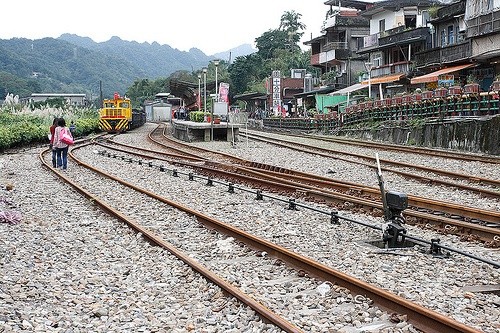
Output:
[60,127,74,145]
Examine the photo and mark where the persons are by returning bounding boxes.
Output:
[49,117,61,168]
[52,118,73,171]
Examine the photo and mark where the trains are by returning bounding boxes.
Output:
[95,91,146,134]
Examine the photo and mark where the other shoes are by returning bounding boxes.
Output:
[53,161,56,168]
[60,167,66,172]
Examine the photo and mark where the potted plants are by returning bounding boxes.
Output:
[214,114,221,124]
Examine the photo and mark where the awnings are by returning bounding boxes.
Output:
[411,64,473,84]
[363,74,405,85]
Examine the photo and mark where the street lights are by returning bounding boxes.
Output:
[198,75,201,111]
[365,63,375,99]
[202,68,208,112]
[212,60,220,101]
